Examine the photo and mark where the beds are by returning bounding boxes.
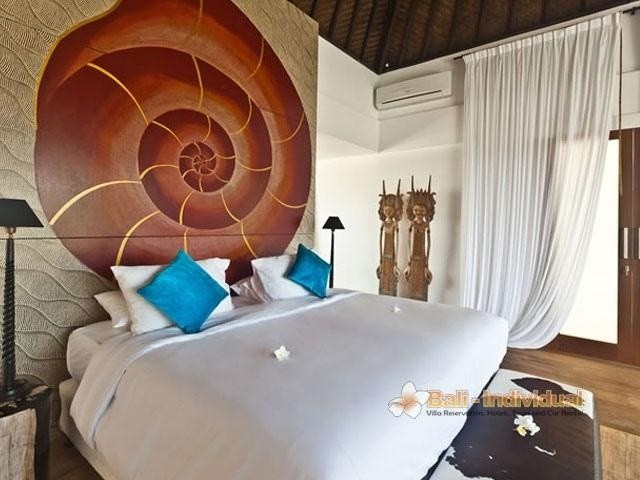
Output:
[59,287,509,480]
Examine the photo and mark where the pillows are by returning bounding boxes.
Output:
[92,243,331,335]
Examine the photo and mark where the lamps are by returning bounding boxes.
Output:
[323,217,345,287]
[1,197,45,402]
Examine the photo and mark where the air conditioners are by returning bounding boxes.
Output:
[375,71,464,121]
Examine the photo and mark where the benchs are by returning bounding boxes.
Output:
[429,368,603,480]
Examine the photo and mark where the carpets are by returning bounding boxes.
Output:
[601,421,640,480]
